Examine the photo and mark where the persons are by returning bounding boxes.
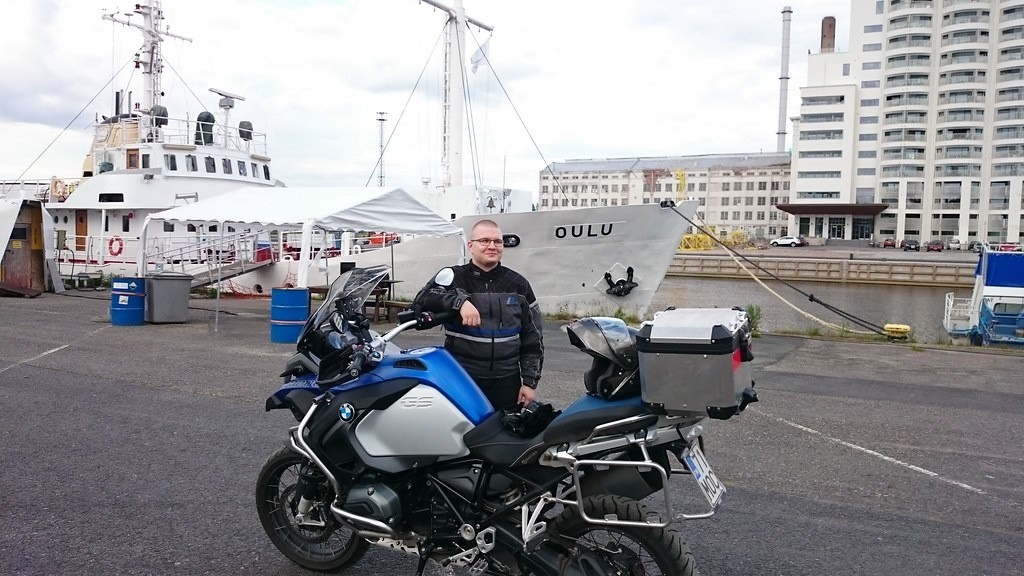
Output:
[410,217,544,412]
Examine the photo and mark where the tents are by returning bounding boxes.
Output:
[139,182,468,332]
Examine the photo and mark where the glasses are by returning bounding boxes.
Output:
[471,238,504,247]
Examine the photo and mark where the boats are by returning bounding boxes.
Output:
[0,1,288,276]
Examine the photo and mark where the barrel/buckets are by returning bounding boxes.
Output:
[270,287,310,344]
[110,277,146,325]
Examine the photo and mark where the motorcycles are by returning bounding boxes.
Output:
[256,264,759,576]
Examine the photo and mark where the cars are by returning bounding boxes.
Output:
[770,236,801,247]
[968,241,984,253]
[884,239,895,248]
[986,241,1022,252]
[927,239,961,252]
[900,240,920,251]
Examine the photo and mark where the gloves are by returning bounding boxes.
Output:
[500,400,562,439]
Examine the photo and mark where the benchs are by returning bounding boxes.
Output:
[363,300,414,323]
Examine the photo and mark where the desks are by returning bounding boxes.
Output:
[307,285,387,324]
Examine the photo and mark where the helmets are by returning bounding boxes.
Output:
[567,316,641,400]
